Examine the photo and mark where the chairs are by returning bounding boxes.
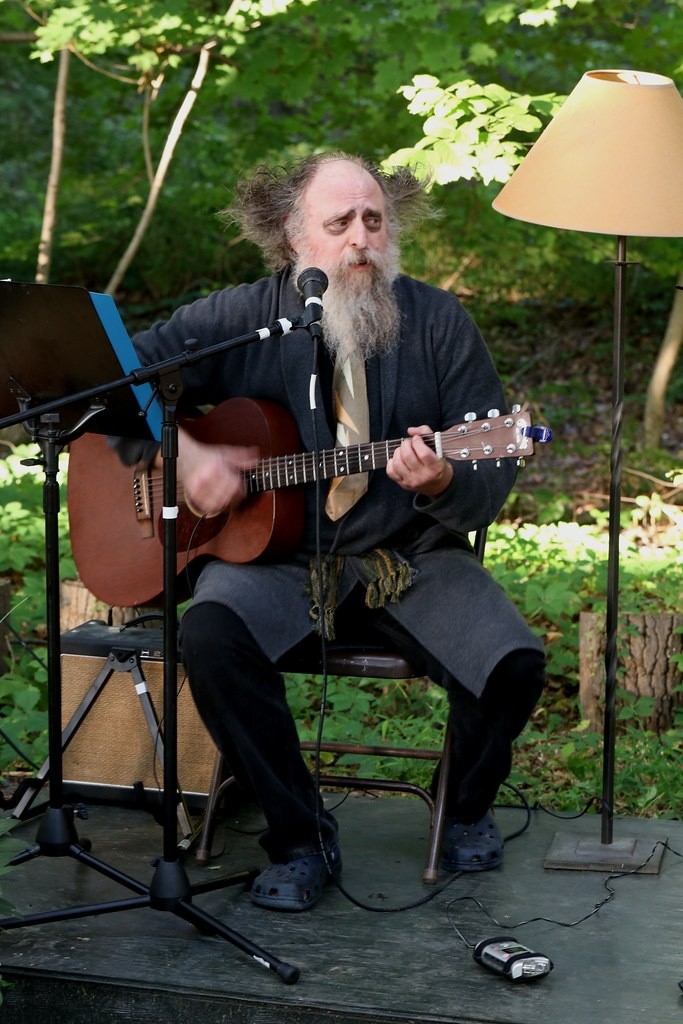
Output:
[191,525,489,889]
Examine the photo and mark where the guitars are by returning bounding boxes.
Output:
[67,396,555,608]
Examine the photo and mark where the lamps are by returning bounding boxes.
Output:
[490,70,682,875]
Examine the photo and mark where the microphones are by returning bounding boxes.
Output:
[297,267,328,341]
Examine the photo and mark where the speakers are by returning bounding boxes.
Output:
[58,618,219,814]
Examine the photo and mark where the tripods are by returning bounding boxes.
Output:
[0,278,302,987]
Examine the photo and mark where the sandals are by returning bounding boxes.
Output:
[438,806,503,869]
[253,818,343,907]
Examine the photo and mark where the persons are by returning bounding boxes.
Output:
[87,153,546,912]
